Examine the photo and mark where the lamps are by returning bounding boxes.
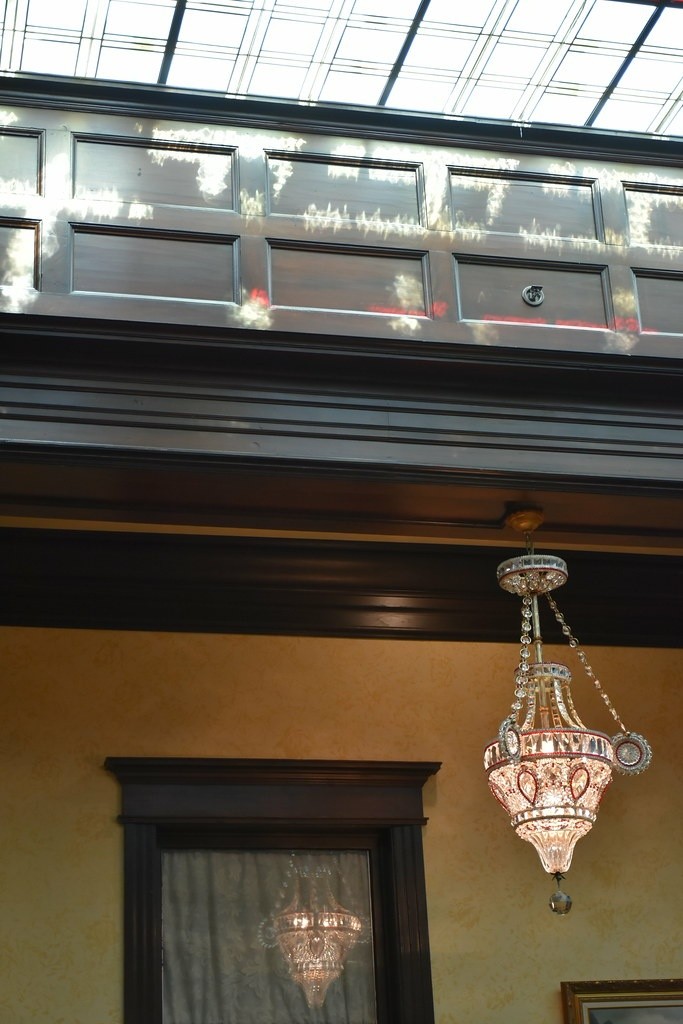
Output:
[257,850,372,1010]
[484,508,652,915]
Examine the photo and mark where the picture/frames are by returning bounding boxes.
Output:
[561,979,683,1024]
